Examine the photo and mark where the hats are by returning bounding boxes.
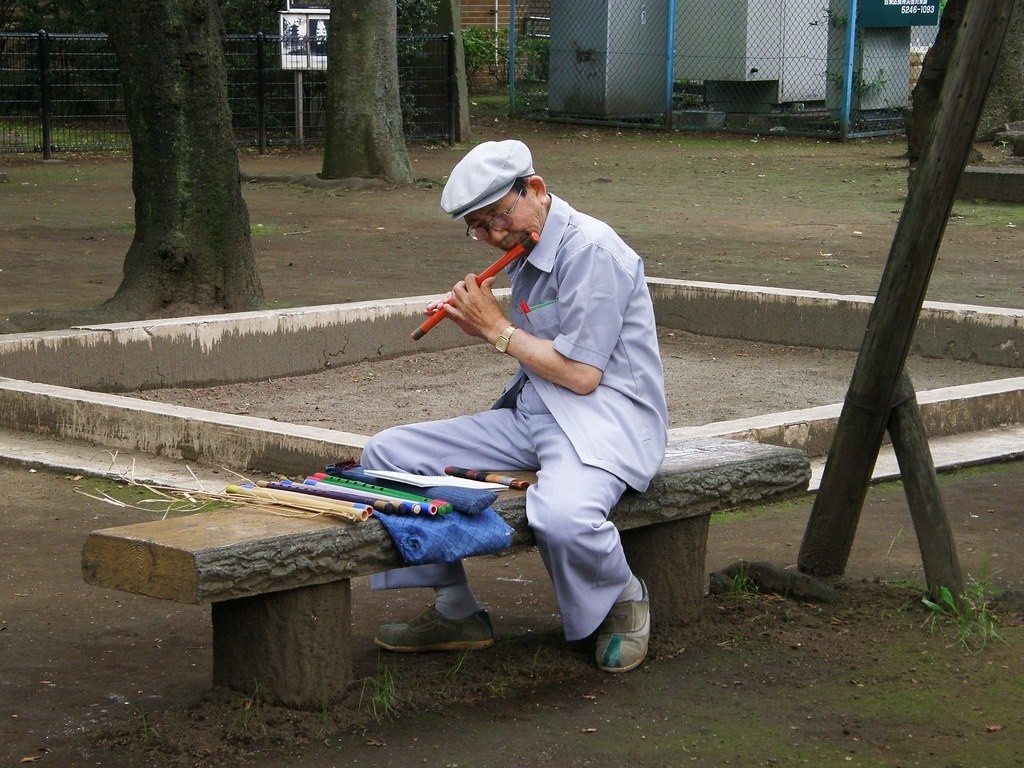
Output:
[439,138,536,222]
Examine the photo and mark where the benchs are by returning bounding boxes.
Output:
[906,166,1024,204]
[80,435,812,705]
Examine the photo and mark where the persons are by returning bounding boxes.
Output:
[358,139,669,670]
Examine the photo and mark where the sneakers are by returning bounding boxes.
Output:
[374,603,494,652]
[595,575,651,672]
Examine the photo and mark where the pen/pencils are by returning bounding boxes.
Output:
[519,300,530,312]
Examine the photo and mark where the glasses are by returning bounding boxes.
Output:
[466,188,525,241]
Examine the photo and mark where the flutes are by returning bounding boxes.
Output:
[225,472,453,522]
[410,232,540,341]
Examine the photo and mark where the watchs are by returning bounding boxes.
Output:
[494,324,519,354]
